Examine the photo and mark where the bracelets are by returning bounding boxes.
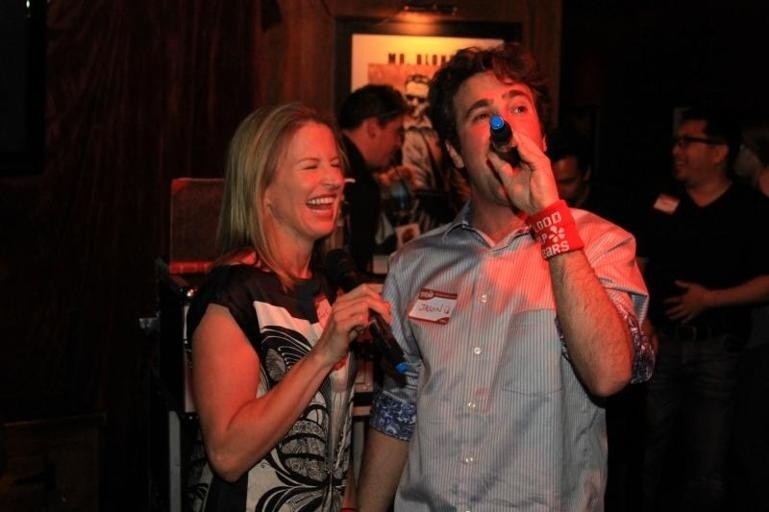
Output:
[524,199,585,260]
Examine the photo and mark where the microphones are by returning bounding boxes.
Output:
[490,113,521,169]
[324,248,409,375]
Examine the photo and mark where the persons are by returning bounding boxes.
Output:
[636,109,769,512]
[399,74,469,239]
[542,124,630,233]
[181,103,393,512]
[331,83,406,283]
[355,45,656,512]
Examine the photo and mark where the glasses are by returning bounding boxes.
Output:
[672,135,710,145]
